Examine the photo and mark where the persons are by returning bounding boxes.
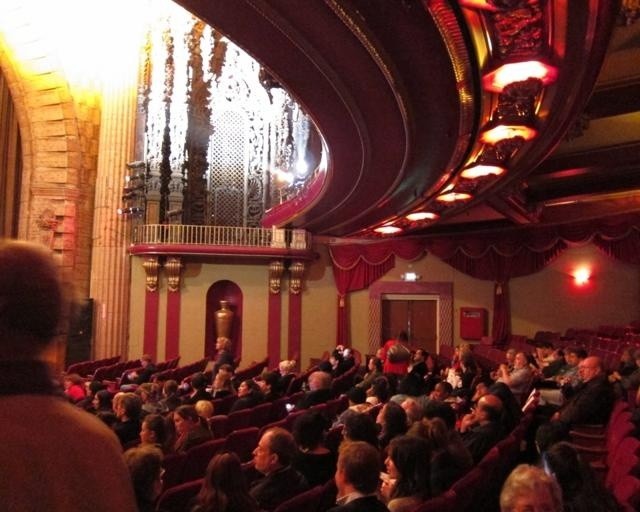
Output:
[0,237,138,512]
[60,327,640,512]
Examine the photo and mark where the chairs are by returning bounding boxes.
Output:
[65,320,639,512]
